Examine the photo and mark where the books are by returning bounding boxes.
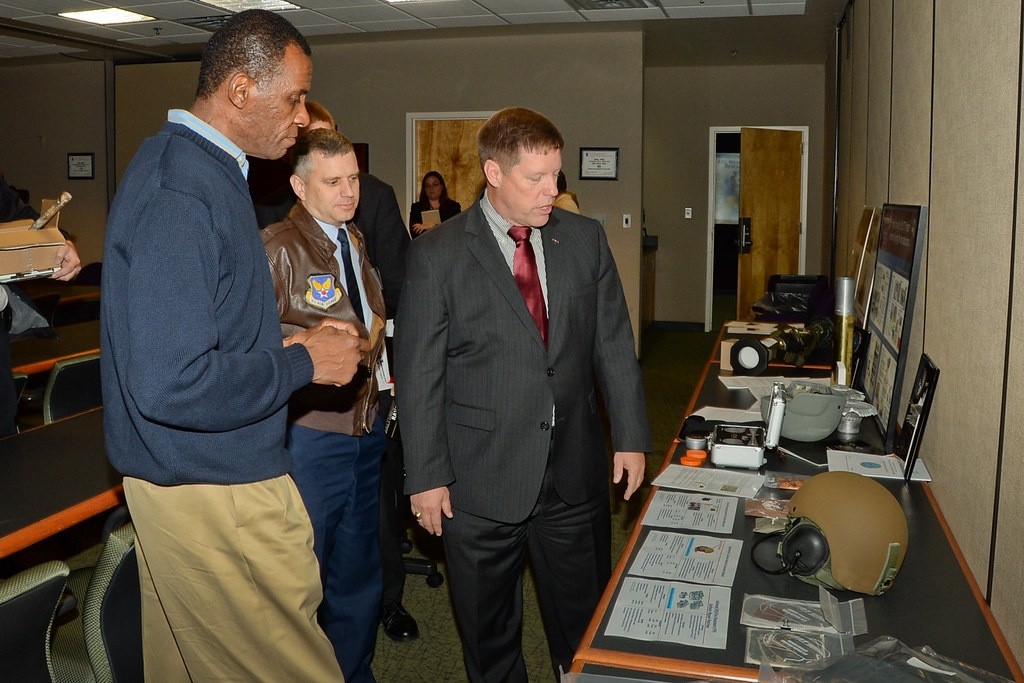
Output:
[421,210,440,225]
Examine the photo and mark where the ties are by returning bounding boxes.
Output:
[337,228,366,329]
[507,226,549,353]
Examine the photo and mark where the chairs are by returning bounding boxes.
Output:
[0,262,142,682]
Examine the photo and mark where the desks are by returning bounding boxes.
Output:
[6,317,100,376]
[10,279,101,303]
[561,320,1024,683]
[1,399,127,559]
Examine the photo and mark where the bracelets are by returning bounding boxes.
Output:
[433,223,438,228]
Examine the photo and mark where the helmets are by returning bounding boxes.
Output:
[777,471,908,596]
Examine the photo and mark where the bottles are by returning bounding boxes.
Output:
[831,277,855,388]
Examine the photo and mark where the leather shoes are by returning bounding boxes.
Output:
[382,603,419,642]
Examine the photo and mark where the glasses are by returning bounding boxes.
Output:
[424,181,440,189]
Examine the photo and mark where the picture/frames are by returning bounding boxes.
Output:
[861,204,928,449]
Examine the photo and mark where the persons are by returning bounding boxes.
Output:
[398,106,647,683]
[102,7,359,683]
[1,99,580,683]
